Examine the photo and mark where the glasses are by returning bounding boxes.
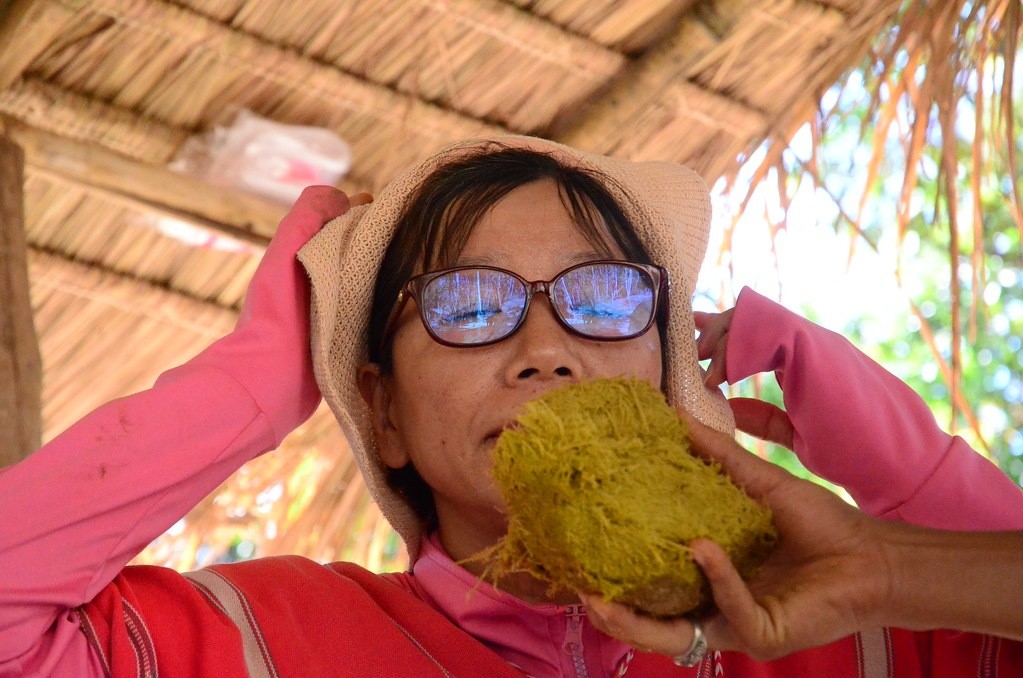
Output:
[372,259,661,349]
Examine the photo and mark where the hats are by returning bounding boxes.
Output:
[297,135,736,576]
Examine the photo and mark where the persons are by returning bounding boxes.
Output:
[0,132,1023,678]
[575,404,1023,669]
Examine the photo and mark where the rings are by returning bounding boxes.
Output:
[672,620,709,668]
